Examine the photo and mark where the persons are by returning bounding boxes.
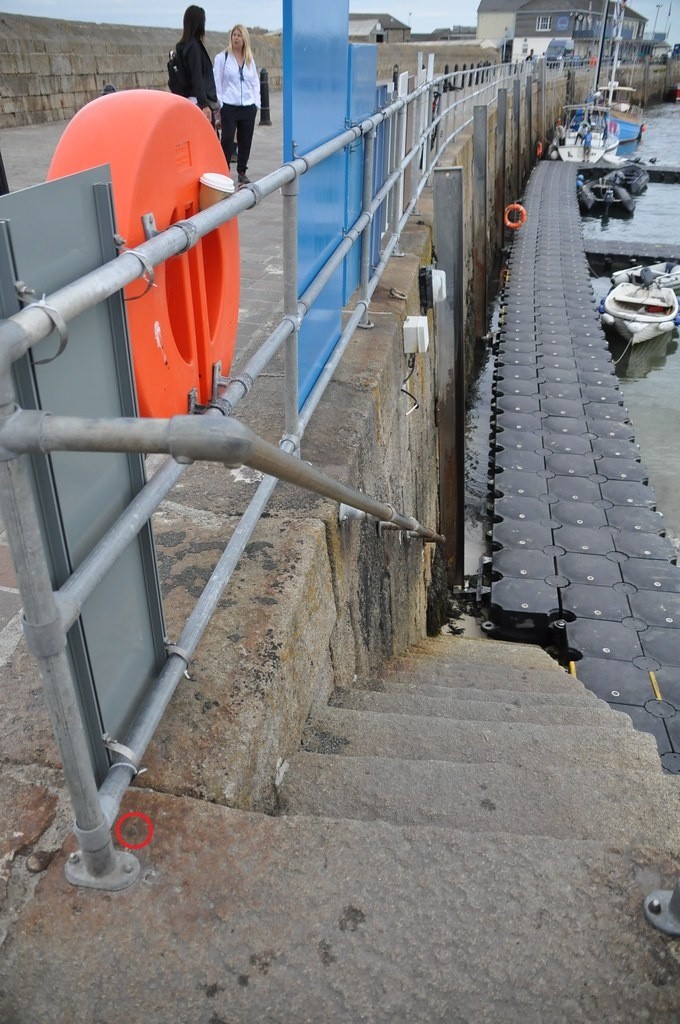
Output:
[581,131,593,162]
[213,23,261,184]
[168,5,221,128]
[575,118,592,145]
[556,125,565,146]
[526,49,534,61]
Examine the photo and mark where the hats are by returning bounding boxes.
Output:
[100,85,116,94]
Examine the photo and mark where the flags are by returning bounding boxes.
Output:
[612,0,626,39]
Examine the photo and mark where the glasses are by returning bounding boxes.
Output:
[239,67,244,81]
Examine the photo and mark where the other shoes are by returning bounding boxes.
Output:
[230,154,237,162]
[238,174,251,184]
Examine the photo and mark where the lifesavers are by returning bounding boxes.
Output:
[503,203,527,228]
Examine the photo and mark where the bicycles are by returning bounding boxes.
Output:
[430,82,463,148]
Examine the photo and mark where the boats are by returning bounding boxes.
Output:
[608,260,680,294]
[599,268,680,348]
[579,161,656,213]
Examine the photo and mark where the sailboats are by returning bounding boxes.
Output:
[546,0,646,161]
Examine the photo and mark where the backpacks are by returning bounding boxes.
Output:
[166,35,193,99]
